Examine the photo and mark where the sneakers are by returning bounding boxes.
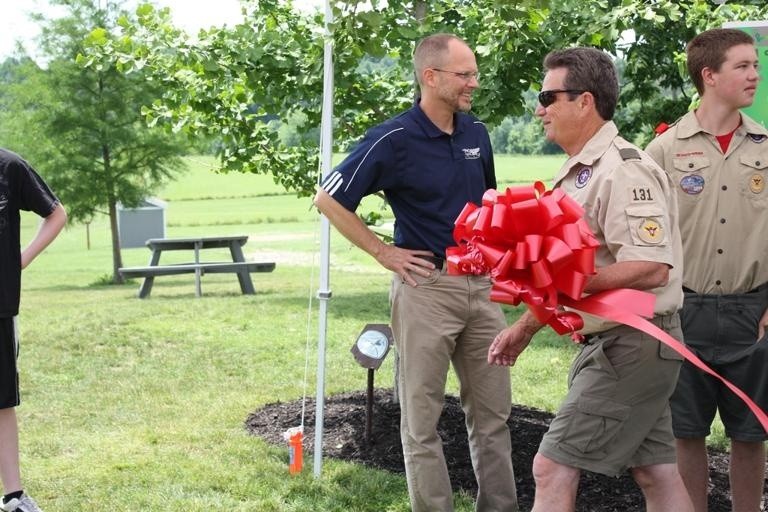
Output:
[2,490,40,512]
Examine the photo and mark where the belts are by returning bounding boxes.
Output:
[415,253,445,270]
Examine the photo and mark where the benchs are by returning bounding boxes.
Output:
[118,234,275,298]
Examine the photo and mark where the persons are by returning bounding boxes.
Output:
[486,47,693,510]
[313,33,520,511]
[642,28,768,511]
[0,150,69,511]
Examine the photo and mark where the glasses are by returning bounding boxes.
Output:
[431,64,481,79]
[538,85,582,106]
